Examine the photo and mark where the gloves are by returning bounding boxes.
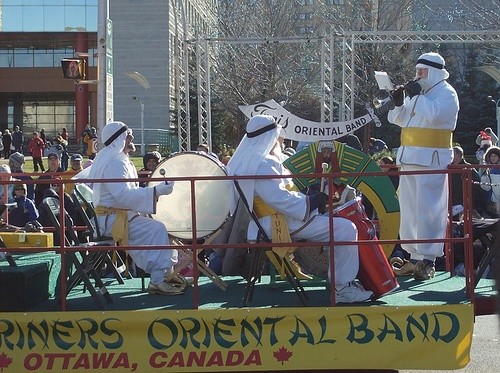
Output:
[390,80,422,107]
[309,192,328,215]
[155,181,174,197]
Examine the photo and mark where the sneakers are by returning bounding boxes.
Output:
[335,282,374,304]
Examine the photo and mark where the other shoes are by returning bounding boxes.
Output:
[476,264,491,279]
[389,256,436,280]
[147,274,190,295]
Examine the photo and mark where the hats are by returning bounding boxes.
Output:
[12,184,27,194]
[47,153,58,159]
[71,153,84,161]
[381,157,394,171]
[9,152,25,167]
[0,164,11,174]
[452,146,463,157]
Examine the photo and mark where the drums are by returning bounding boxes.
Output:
[320,197,400,301]
[143,150,233,242]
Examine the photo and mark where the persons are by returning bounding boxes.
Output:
[0,116,500,305]
[387,54,459,277]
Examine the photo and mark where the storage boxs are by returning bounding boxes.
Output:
[0,230,53,248]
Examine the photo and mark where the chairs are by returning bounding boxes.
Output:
[43,184,133,311]
[233,180,311,308]
